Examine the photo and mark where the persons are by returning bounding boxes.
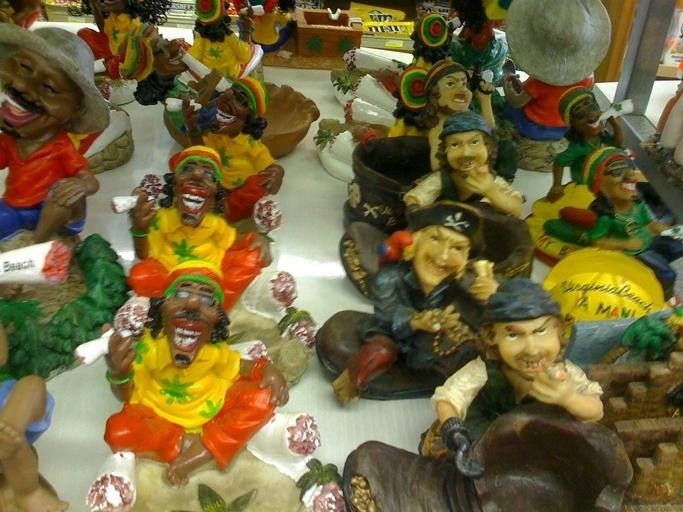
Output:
[331,200,499,405]
[0,327,69,512]
[0,29,110,254]
[417,278,603,479]
[103,260,289,488]
[111,145,272,313]
[165,76,284,219]
[1,0,682,209]
[376,111,522,230]
[581,146,681,301]
